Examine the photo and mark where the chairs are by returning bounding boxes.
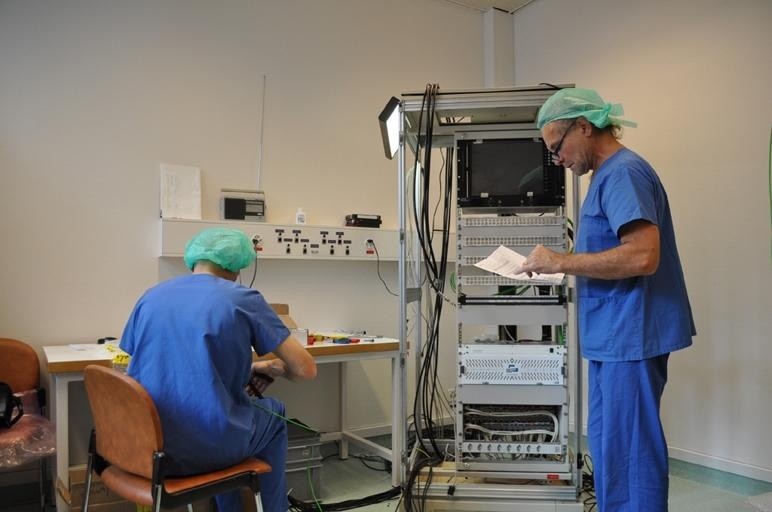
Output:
[1,338,56,512]
[83,364,273,512]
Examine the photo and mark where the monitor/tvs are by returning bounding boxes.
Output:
[457,139,566,209]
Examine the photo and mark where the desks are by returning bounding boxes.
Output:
[42,331,410,512]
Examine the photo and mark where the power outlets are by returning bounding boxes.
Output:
[250,233,265,246]
[365,239,375,249]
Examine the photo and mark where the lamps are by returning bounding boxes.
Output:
[378,95,402,161]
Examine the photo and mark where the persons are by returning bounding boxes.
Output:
[116,224,321,510]
[509,83,700,511]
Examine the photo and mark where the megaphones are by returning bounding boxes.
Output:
[220,188,266,220]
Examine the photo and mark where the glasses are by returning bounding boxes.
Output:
[551,120,576,160]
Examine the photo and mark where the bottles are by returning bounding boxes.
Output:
[296,207,306,224]
